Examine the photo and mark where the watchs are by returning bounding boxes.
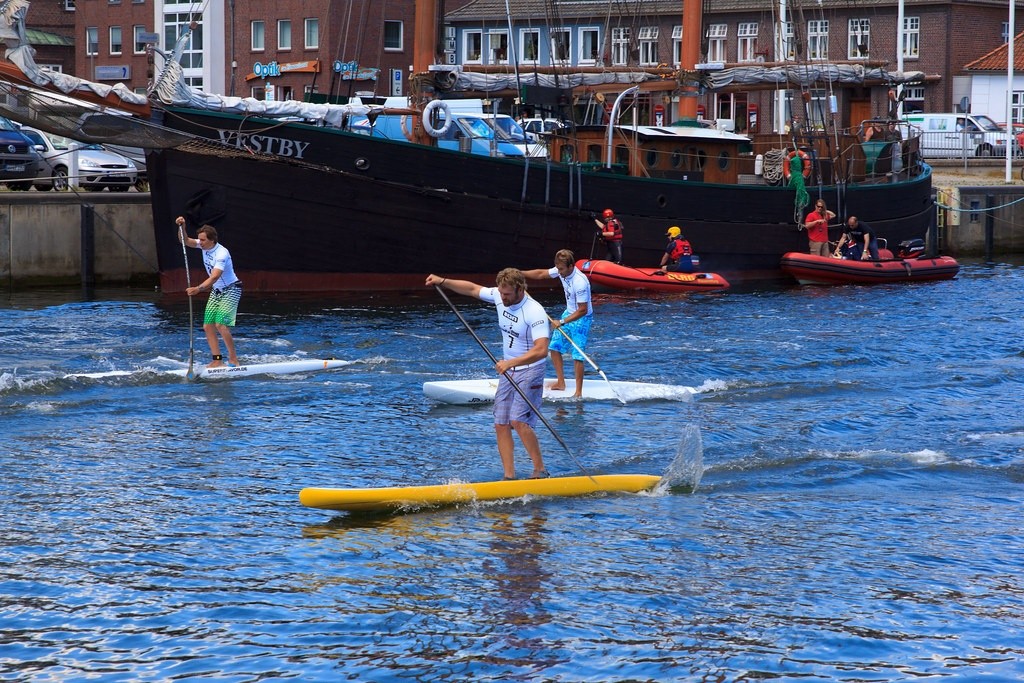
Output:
[198,285,204,292]
[560,319,565,326]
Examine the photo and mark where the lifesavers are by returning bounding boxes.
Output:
[400,104,413,141]
[422,99,452,137]
[783,150,811,180]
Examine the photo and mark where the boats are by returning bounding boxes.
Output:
[573,257,733,293]
[778,247,960,283]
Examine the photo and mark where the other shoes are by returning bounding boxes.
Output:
[527,468,550,479]
[502,475,518,482]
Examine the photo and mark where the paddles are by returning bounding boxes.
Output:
[178,216,195,382]
[433,283,589,474]
[546,313,627,405]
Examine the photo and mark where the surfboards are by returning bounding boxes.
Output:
[422,376,698,405]
[296,474,665,510]
[63,359,349,381]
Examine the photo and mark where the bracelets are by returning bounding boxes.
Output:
[439,278,446,285]
[863,250,867,254]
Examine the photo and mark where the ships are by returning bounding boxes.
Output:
[144,0,938,297]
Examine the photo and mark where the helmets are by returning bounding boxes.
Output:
[665,227,681,237]
[602,209,613,219]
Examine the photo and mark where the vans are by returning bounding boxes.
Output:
[901,113,1020,157]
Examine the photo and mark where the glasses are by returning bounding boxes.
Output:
[816,204,824,208]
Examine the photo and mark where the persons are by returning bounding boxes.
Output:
[806,199,836,256]
[519,249,595,399]
[834,217,879,260]
[957,119,966,131]
[591,209,623,264]
[661,226,697,273]
[866,116,902,142]
[426,268,549,481]
[514,111,527,123]
[176,216,241,369]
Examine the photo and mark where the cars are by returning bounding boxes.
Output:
[0,116,149,194]
[990,122,1024,151]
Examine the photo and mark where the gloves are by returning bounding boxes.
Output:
[597,231,603,238]
[658,264,664,267]
[590,211,596,219]
